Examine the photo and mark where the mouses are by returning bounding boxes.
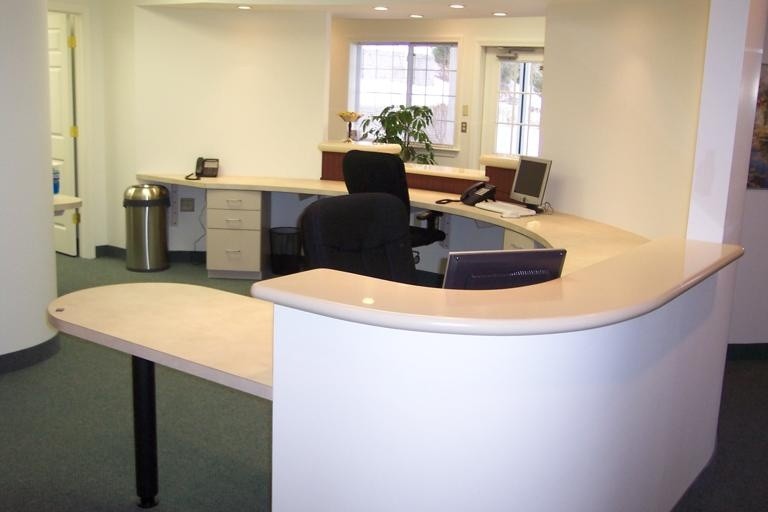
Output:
[501,212,520,218]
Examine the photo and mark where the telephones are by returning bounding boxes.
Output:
[461,181,497,206]
[196,157,219,177]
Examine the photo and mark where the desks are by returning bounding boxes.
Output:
[46,169,648,512]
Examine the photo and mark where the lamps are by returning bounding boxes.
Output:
[338,111,364,145]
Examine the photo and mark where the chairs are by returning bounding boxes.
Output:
[297,149,445,296]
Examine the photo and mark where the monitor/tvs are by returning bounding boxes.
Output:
[442,248,567,290]
[509,155,552,213]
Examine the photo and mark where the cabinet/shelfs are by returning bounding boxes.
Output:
[206,187,273,278]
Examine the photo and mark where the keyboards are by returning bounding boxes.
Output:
[475,199,536,216]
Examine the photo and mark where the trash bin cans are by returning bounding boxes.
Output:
[124,184,169,272]
